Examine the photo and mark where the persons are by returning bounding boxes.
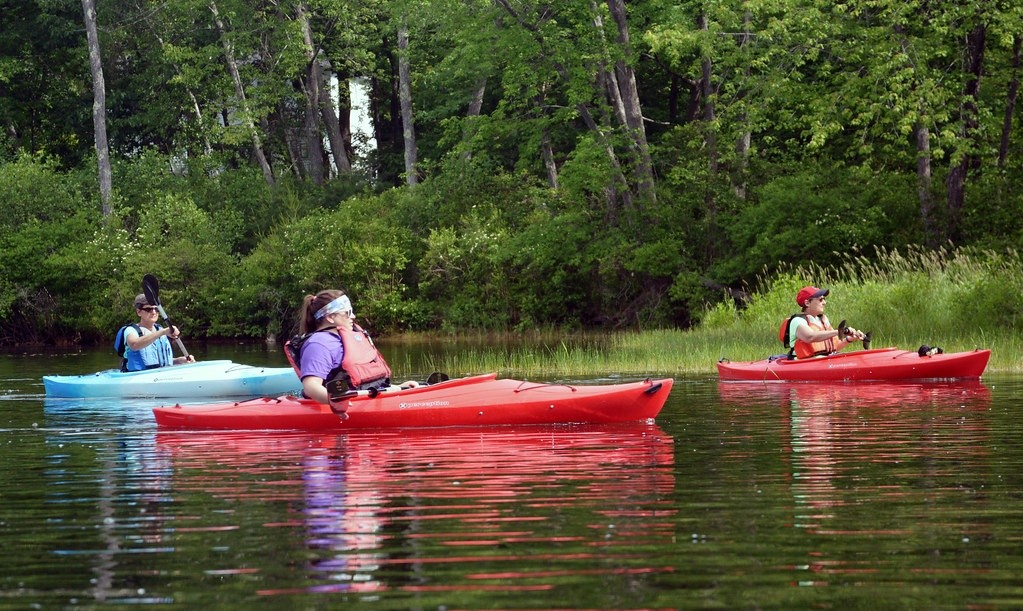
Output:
[789,286,865,360]
[123,294,195,372]
[299,289,419,420]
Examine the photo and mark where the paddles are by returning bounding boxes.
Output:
[838,319,874,351]
[326,372,449,414]
[141,274,191,361]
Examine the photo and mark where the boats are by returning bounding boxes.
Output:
[717,345,992,383]
[153,371,674,436]
[43,359,304,399]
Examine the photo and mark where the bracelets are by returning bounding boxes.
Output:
[846,337,853,343]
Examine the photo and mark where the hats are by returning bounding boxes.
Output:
[797,286,829,306]
[135,294,159,305]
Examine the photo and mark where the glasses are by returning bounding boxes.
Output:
[334,307,352,318]
[807,298,825,302]
[140,307,159,312]
